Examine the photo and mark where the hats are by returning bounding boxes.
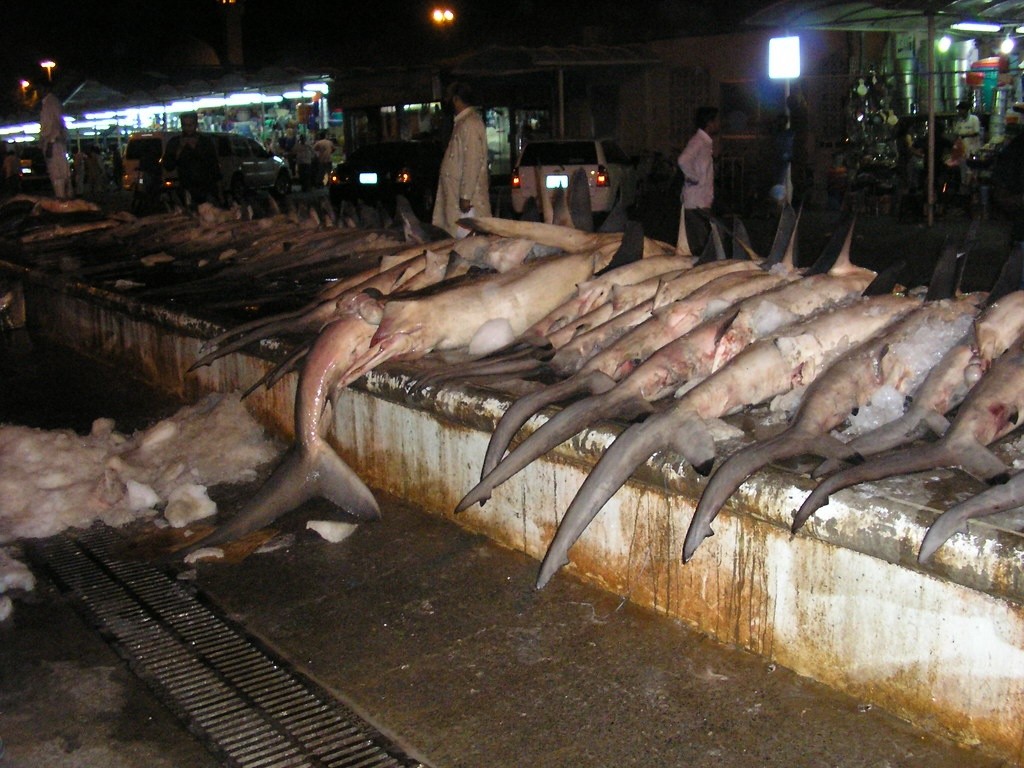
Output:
[957,102,969,108]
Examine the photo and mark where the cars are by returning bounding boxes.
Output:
[328,140,437,224]
[508,139,637,219]
[17,146,74,188]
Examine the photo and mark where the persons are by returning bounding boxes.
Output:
[890,115,925,217]
[0,142,127,195]
[766,110,796,208]
[258,122,345,195]
[952,102,983,206]
[743,121,779,212]
[676,104,723,255]
[34,76,76,200]
[994,119,1023,253]
[161,109,227,210]
[430,78,495,241]
[912,121,960,222]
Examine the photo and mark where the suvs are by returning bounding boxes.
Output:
[120,129,292,204]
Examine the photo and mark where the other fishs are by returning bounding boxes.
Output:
[1,94,1024,593]
